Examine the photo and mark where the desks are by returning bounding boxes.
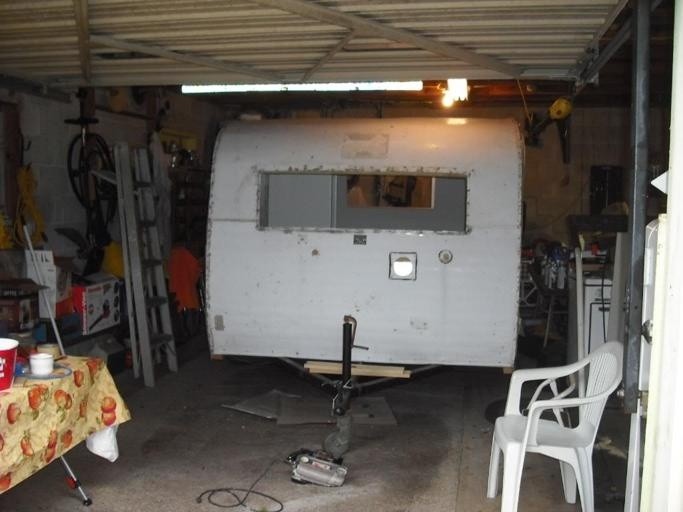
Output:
[0,354,116,507]
[523,258,571,346]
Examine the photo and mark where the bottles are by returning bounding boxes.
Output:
[541,253,567,290]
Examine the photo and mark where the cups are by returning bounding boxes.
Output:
[0,337,19,392]
[29,353,53,375]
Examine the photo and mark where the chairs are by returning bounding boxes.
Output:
[485,341,624,512]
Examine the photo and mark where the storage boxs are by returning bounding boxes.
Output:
[1,248,123,335]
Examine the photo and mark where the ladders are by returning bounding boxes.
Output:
[113,140,179,388]
